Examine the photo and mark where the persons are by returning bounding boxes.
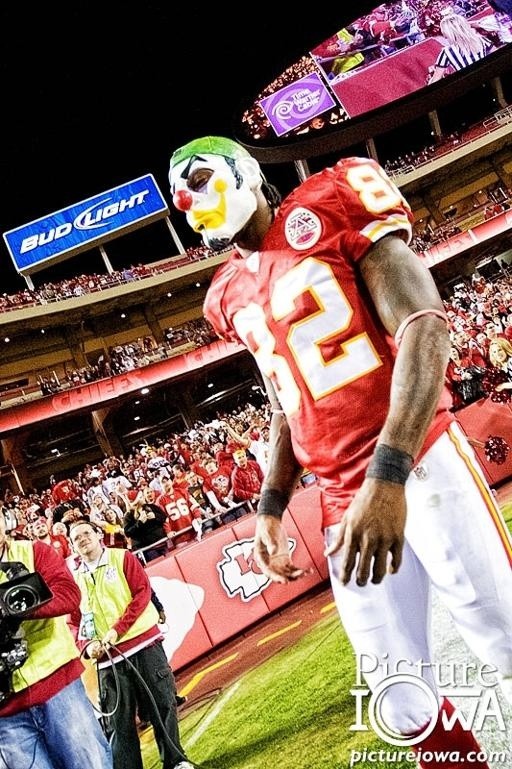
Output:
[240,0,512,140]
[2,129,512,769]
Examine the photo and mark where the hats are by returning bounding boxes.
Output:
[168,135,251,168]
[126,489,143,507]
